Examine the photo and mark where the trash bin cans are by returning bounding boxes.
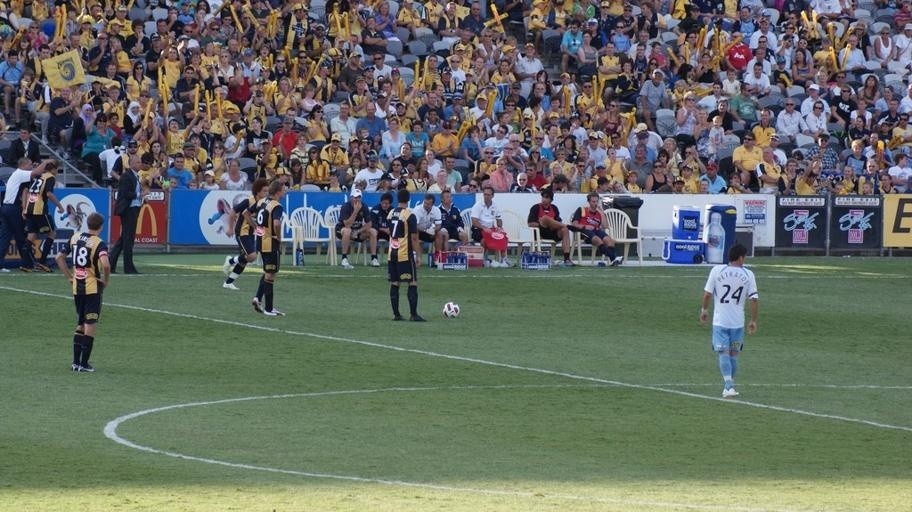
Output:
[704,204,737,264]
[601,195,644,259]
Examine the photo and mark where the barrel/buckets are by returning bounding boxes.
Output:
[671,206,700,240]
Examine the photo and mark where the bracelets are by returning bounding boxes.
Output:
[701,307,707,313]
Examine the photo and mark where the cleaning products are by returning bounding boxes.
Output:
[705,213,726,264]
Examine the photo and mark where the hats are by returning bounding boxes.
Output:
[321,51,407,107]
[588,18,597,25]
[809,84,819,91]
[350,189,361,196]
[452,41,534,100]
[109,0,191,41]
[521,111,647,168]
[245,49,252,55]
[905,24,912,30]
[331,132,377,160]
[600,1,609,7]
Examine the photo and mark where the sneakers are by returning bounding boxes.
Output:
[610,256,623,265]
[253,297,263,311]
[393,317,402,321]
[19,263,51,272]
[341,258,349,264]
[568,225,578,231]
[564,260,574,265]
[72,360,94,372]
[721,388,737,397]
[263,309,285,316]
[502,258,515,267]
[223,256,232,273]
[370,259,379,266]
[223,281,239,290]
[409,316,423,321]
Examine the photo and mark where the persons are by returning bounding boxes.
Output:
[387,190,428,322]
[700,244,759,398]
[253,180,288,319]
[54,211,111,374]
[2,2,912,288]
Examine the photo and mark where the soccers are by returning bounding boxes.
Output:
[442,302,460,318]
